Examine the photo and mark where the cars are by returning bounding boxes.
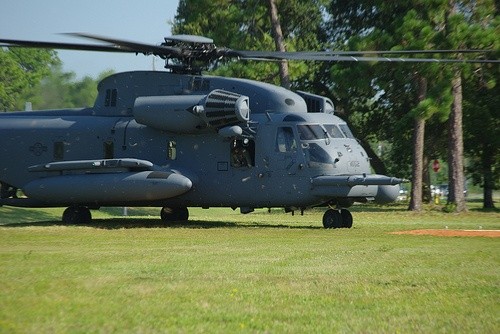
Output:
[393,182,469,204]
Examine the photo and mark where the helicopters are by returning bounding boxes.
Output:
[0,27,500,233]
